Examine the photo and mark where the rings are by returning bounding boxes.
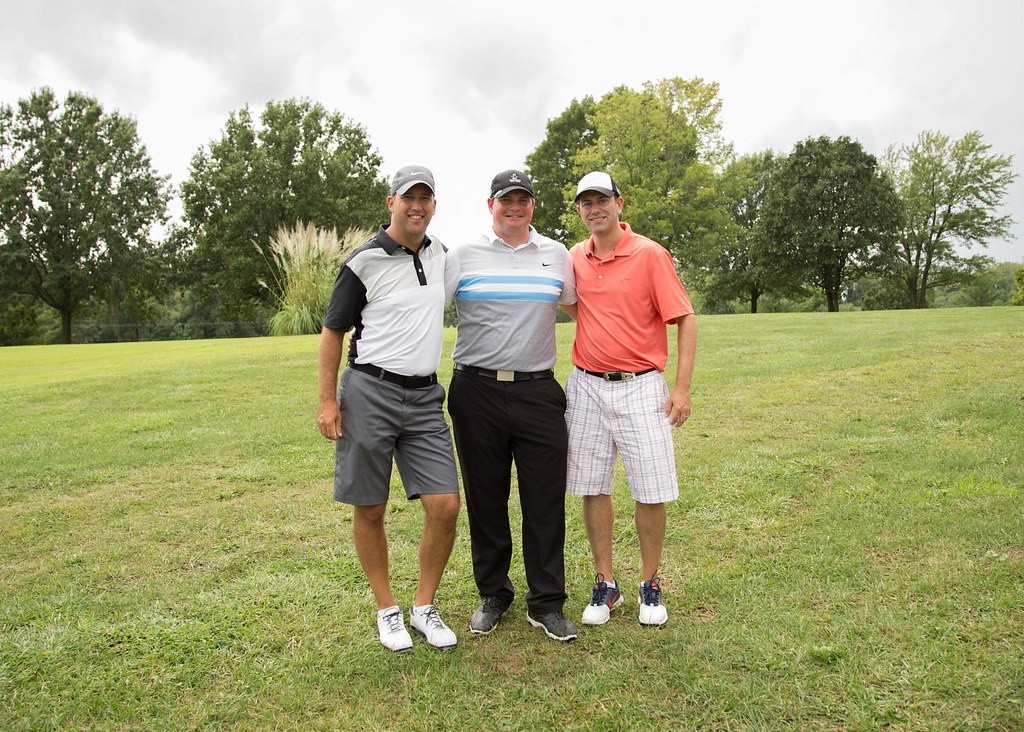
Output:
[685,416,688,418]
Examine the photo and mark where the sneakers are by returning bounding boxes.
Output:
[470,595,508,634]
[581,572,625,625]
[377,606,413,653]
[409,606,457,649]
[526,608,577,640]
[638,570,668,626]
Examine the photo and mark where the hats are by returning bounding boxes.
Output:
[574,169,621,204]
[490,170,535,198]
[390,166,436,197]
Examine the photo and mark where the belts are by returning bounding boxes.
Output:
[349,360,438,389]
[454,362,552,382]
[576,365,656,380]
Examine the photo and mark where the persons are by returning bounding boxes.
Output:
[315,165,461,651]
[565,172,698,626]
[446,170,578,641]
